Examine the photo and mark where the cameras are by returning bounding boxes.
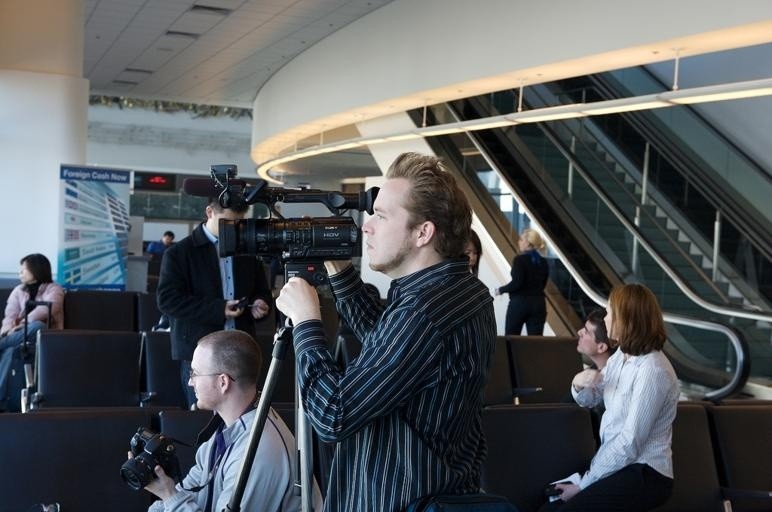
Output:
[120,427,177,492]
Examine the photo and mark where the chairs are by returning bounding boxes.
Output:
[0,282,772,512]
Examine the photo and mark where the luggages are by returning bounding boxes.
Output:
[1,300,51,411]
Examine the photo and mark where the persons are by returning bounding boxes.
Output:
[146,231,174,261]
[494,229,550,336]
[538,284,679,511]
[576,308,619,455]
[0,254,66,413]
[127,330,324,512]
[463,230,481,278]
[274,152,497,511]
[156,196,272,409]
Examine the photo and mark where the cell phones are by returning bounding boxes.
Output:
[545,481,573,497]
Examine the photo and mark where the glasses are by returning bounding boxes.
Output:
[189,371,237,385]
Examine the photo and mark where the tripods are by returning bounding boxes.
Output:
[222,262,327,512]
